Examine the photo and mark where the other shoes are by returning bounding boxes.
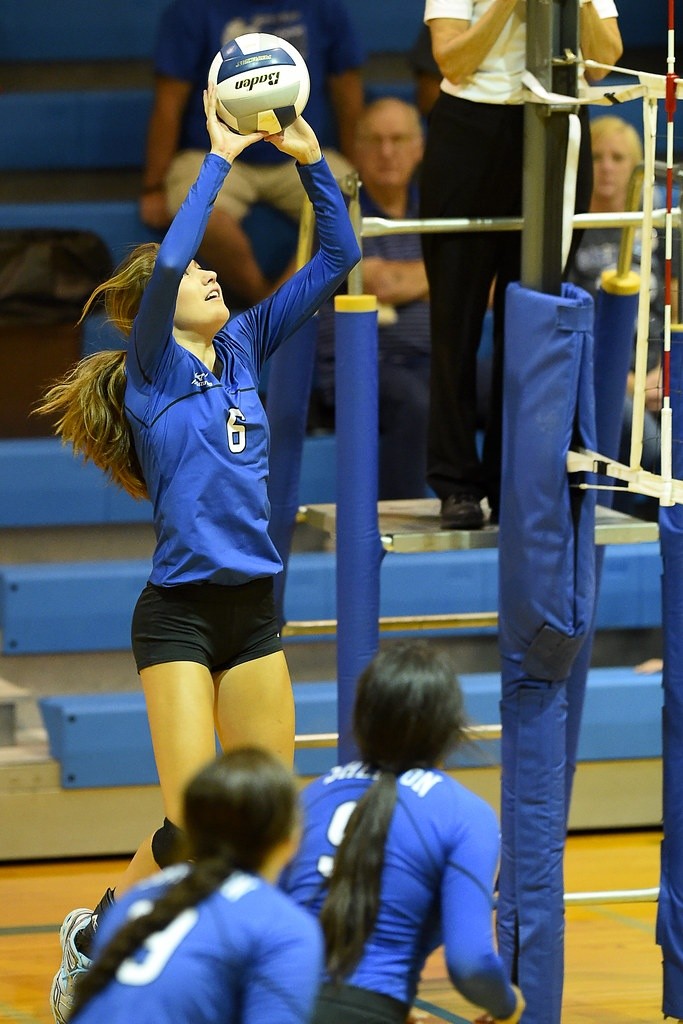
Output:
[490,509,501,523]
[441,491,484,530]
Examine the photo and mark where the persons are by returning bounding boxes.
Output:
[26,0,683,1024]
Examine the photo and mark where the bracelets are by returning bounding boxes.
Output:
[141,180,165,195]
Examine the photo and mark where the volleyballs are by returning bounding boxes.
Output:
[207,32,311,139]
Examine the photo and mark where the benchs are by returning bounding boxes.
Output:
[0,1,683,864]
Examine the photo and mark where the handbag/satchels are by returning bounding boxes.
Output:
[3,225,114,325]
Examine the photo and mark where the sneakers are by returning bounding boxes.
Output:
[49,908,93,1024]
